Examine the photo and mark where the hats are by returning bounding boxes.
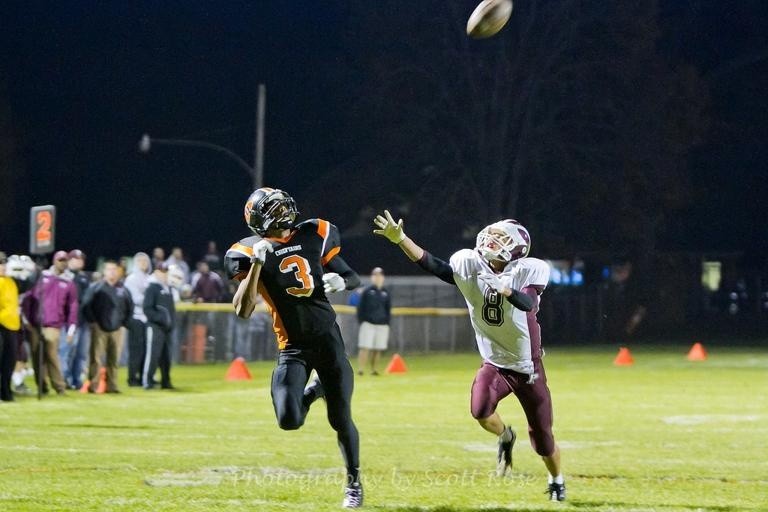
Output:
[68,249,85,261]
[54,251,69,261]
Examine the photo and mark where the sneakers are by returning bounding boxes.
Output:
[546,480,567,502]
[5,377,174,402]
[497,427,516,478]
[342,483,363,506]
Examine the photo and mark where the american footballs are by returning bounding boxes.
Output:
[467,1,513,37]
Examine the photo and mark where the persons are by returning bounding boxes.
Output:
[344,283,363,358]
[371,209,568,503]
[354,266,392,377]
[224,186,365,511]
[0,240,278,401]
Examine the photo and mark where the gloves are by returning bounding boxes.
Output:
[323,272,347,294]
[477,269,507,295]
[372,209,408,247]
[253,239,276,260]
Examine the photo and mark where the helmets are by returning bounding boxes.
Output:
[475,217,532,265]
[244,186,301,234]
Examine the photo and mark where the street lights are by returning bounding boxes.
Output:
[135,84,266,193]
